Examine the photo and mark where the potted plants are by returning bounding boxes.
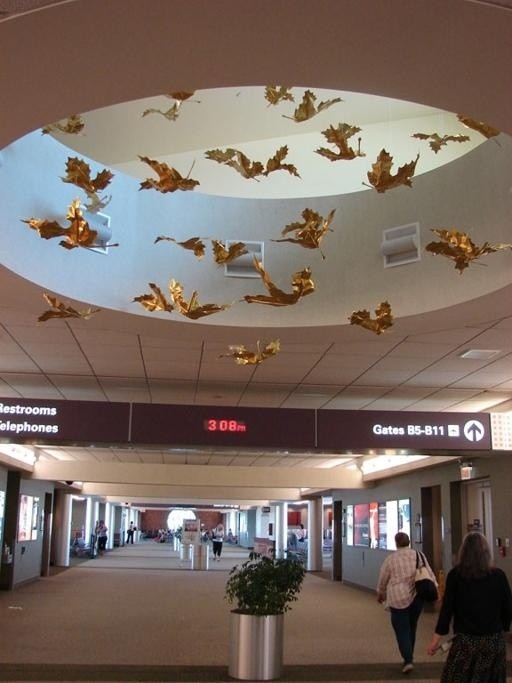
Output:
[221,547,308,682]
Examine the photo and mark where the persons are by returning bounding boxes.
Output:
[154,528,233,545]
[126,520,134,543]
[212,521,225,561]
[427,532,511,683]
[96,519,107,555]
[377,532,441,672]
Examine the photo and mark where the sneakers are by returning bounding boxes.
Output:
[402,663,412,672]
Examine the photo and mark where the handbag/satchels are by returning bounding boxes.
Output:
[413,566,440,602]
